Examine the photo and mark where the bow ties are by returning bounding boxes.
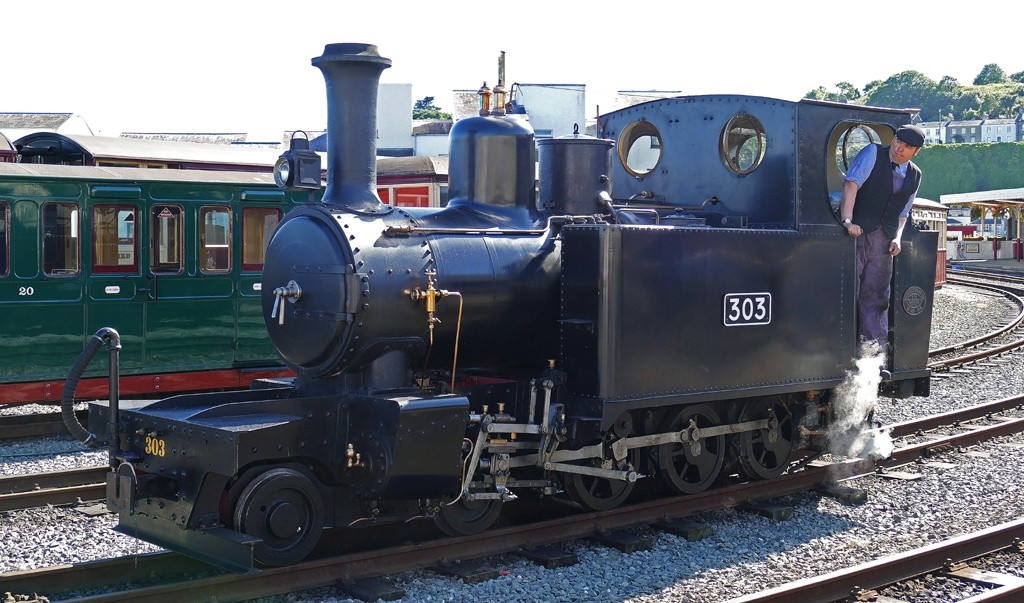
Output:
[891,162,900,171]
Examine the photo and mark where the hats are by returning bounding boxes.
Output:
[897,124,924,147]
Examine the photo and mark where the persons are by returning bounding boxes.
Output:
[840,125,925,380]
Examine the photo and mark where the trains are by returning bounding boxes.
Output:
[59,42,941,572]
[0,155,949,407]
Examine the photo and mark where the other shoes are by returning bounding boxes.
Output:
[879,369,891,380]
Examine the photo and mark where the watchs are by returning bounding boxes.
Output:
[841,218,852,224]
